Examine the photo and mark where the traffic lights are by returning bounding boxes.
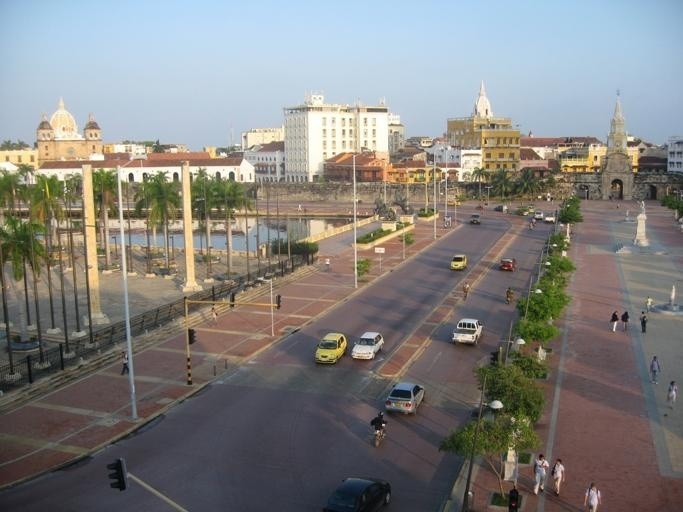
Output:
[189,329,196,344]
[105,455,128,491]
[507,488,517,512]
[276,295,282,308]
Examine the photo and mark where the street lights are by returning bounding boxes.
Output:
[534,249,551,280]
[462,373,503,511]
[376,155,428,214]
[432,145,446,240]
[115,153,150,425]
[499,321,525,367]
[352,147,374,292]
[543,233,559,254]
[525,274,543,318]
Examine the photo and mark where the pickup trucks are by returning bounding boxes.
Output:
[452,318,483,345]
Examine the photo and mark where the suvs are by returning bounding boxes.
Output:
[471,213,481,224]
[314,332,348,365]
[501,257,519,271]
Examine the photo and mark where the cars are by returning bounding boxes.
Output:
[446,198,460,205]
[521,204,554,224]
[351,332,384,360]
[384,382,426,415]
[323,477,391,512]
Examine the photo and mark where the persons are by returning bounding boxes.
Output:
[121,352,129,375]
[584,483,601,512]
[650,357,661,384]
[506,287,513,299]
[325,257,330,271]
[611,297,654,333]
[533,455,549,495]
[371,412,387,435]
[211,307,217,323]
[551,459,565,496]
[667,381,677,408]
[463,282,471,297]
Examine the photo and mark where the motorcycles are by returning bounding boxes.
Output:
[506,289,514,304]
[525,219,536,231]
[372,421,388,446]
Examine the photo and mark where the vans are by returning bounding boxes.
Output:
[450,255,467,271]
[494,204,508,211]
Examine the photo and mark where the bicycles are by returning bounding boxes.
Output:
[464,287,470,299]
